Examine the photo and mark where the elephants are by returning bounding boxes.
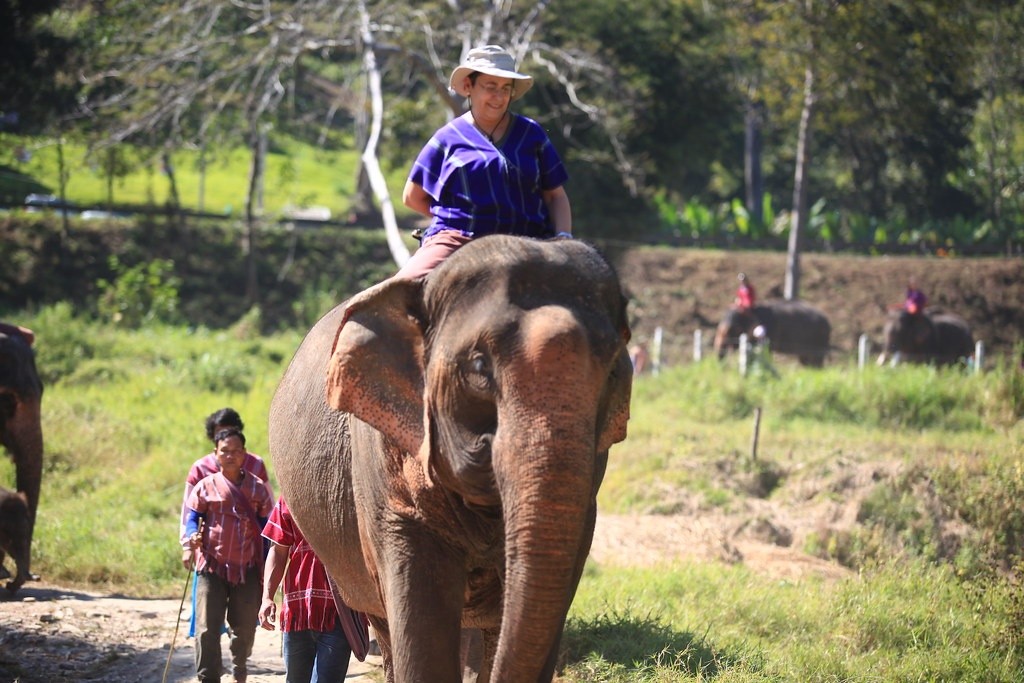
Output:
[881,312,976,374]
[266,234,635,683]
[0,324,45,590]
[713,305,833,371]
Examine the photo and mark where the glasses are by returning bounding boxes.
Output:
[476,79,515,98]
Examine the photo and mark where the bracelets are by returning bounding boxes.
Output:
[554,231,572,238]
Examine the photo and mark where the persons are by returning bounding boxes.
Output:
[626,337,654,376]
[257,495,357,683]
[903,277,938,341]
[731,273,760,339]
[397,44,581,280]
[177,407,273,683]
[748,323,772,365]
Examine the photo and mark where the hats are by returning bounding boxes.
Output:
[450,45,534,102]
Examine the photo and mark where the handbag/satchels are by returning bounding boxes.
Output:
[255,534,272,593]
[325,566,370,662]
